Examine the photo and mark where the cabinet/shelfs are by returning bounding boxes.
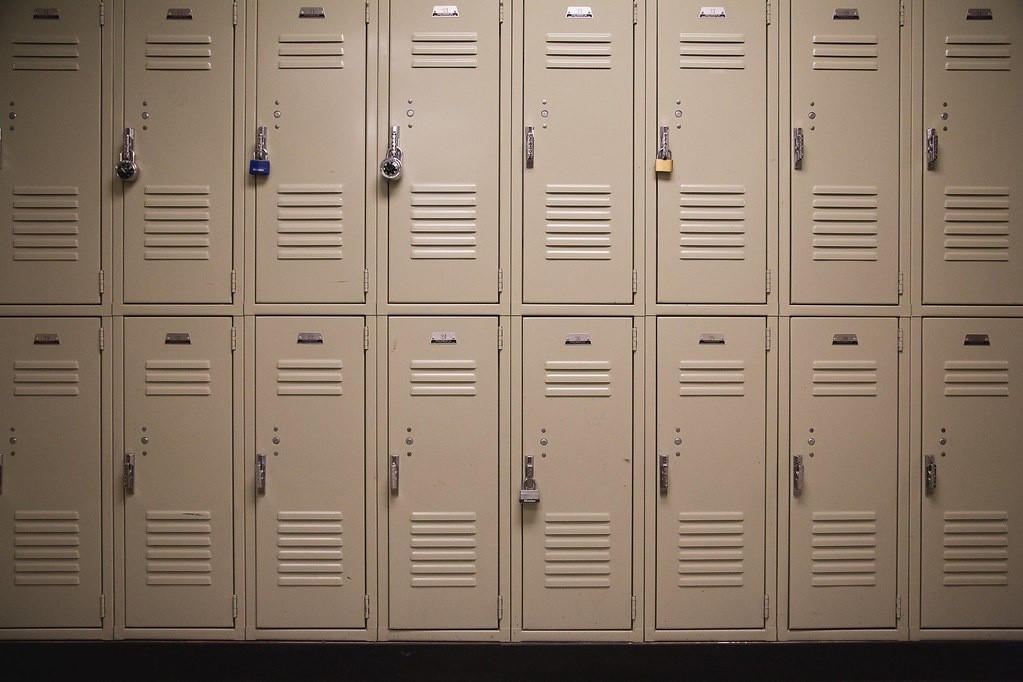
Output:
[0,0,1021,648]
